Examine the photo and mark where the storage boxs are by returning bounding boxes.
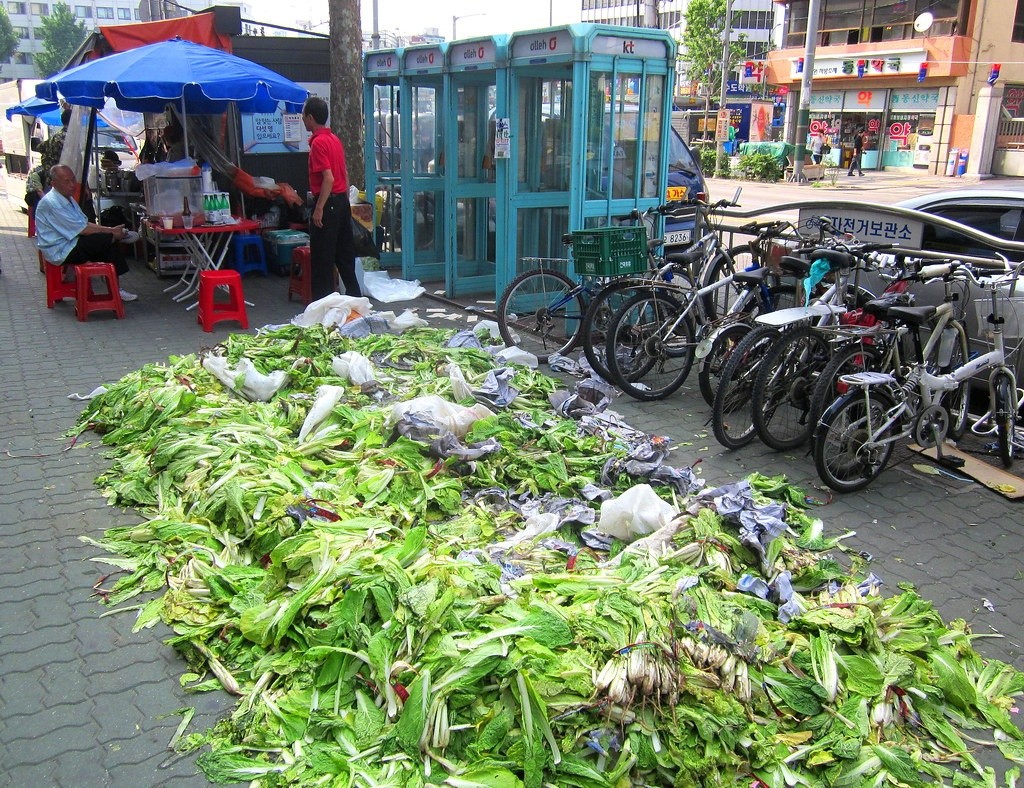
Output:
[844,142,855,148]
[845,151,850,158]
[160,253,206,268]
[244,194,289,229]
[844,161,849,169]
[263,229,311,267]
[148,174,205,227]
[194,193,232,218]
[844,128,851,134]
[571,225,648,277]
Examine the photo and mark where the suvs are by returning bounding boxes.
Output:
[370,100,709,265]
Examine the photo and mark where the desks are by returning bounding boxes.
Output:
[153,219,261,311]
[351,203,373,258]
[143,214,243,301]
[101,190,145,262]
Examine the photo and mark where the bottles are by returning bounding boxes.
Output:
[182,196,191,217]
[203,193,229,225]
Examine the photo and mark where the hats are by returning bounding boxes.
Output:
[858,127,865,133]
[162,126,180,139]
[817,129,824,134]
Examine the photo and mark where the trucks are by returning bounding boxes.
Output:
[0,80,48,174]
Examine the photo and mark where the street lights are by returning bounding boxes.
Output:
[452,13,488,41]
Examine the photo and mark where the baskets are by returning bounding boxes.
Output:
[973,297,1024,339]
[766,241,800,274]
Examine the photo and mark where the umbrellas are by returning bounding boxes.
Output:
[5,95,59,122]
[36,35,312,157]
[40,109,140,127]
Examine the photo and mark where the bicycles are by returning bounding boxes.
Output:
[497,191,1023,495]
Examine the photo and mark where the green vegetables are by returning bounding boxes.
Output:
[58,330,1024,788]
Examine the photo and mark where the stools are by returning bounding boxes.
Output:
[75,262,126,322]
[28,206,35,238]
[198,270,250,332]
[228,233,268,279]
[43,258,95,308]
[287,246,312,304]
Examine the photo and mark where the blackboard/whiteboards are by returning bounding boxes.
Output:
[241,82,331,154]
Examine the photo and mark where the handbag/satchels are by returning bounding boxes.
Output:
[820,144,831,154]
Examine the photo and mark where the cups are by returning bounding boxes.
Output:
[182,216,193,229]
[162,217,173,229]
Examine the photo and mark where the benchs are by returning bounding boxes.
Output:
[785,155,825,180]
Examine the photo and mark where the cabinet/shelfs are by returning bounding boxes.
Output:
[142,219,206,280]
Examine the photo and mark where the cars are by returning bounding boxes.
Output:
[767,191,1023,355]
[86,127,141,192]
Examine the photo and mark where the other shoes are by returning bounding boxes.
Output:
[847,173,854,176]
[859,173,864,176]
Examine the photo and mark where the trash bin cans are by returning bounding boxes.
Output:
[350,202,373,235]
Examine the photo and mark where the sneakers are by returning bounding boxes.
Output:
[119,288,138,302]
[116,231,140,243]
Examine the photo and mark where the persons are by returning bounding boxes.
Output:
[100,152,121,172]
[163,126,185,162]
[24,167,53,207]
[35,164,139,300]
[847,129,865,176]
[303,97,361,298]
[811,129,825,163]
[37,109,71,165]
